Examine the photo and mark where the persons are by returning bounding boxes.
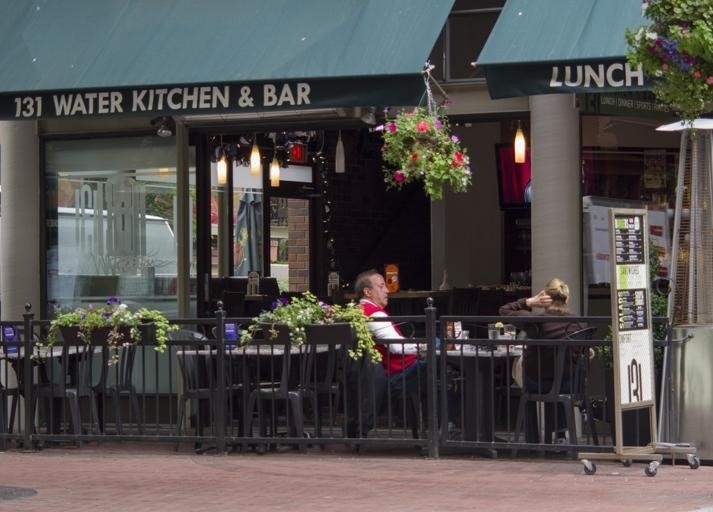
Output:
[498,278,596,458]
[352,270,461,437]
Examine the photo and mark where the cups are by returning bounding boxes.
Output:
[488,323,516,351]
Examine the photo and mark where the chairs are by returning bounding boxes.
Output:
[1,285,600,458]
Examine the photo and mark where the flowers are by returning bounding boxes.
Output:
[624,1,713,137]
[371,96,474,203]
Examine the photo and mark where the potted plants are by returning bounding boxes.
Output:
[595,236,668,447]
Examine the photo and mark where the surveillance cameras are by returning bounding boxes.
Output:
[151,116,174,138]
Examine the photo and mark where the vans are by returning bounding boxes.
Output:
[57,204,176,274]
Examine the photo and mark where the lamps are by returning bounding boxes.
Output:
[513,113,527,164]
[271,144,280,188]
[215,135,228,185]
[156,121,173,138]
[361,105,376,124]
[249,131,262,178]
[334,126,346,174]
